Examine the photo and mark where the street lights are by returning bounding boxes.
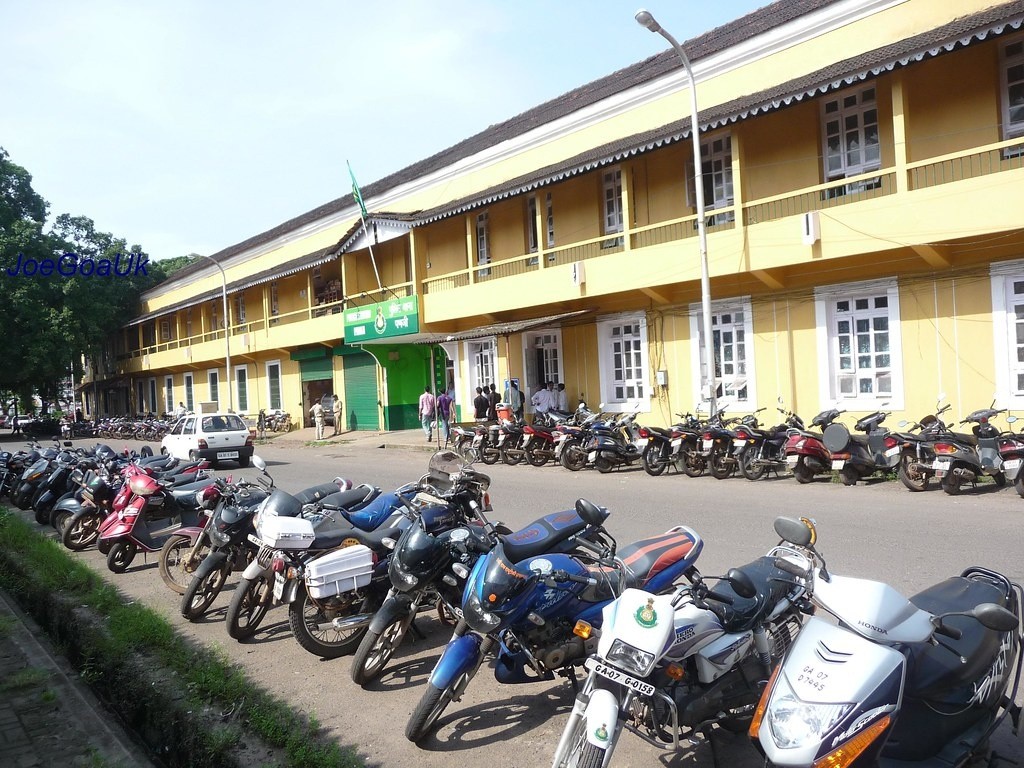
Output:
[635,8,719,416]
[188,253,232,411]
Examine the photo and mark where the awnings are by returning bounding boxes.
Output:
[413,306,599,446]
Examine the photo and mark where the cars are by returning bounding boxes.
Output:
[0,413,32,429]
[161,414,255,468]
[312,391,335,426]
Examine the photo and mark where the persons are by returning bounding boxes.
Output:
[474,381,526,421]
[445,382,456,423]
[27,411,32,420]
[75,408,83,421]
[436,387,456,442]
[331,395,343,435]
[309,398,330,440]
[11,414,21,438]
[419,386,436,442]
[59,414,74,438]
[532,381,569,420]
[175,402,187,423]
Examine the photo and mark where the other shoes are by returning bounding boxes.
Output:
[426,433,432,442]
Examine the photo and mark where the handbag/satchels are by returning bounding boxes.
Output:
[430,420,437,430]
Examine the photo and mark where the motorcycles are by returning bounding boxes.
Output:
[0,435,216,551]
[550,497,819,768]
[21,412,185,443]
[446,399,624,472]
[402,488,710,744]
[994,415,1024,499]
[639,395,804,483]
[882,391,954,492]
[256,409,291,434]
[745,513,1024,768]
[159,454,606,690]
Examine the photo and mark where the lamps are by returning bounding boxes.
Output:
[380,285,403,299]
[804,212,821,246]
[574,261,586,285]
[342,295,359,309]
[361,290,379,304]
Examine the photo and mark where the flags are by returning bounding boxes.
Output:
[348,164,367,217]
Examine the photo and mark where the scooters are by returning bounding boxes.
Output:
[96,445,233,572]
[584,399,648,473]
[931,391,1008,496]
[782,401,891,486]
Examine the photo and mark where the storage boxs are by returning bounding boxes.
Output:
[305,542,373,599]
[428,449,474,490]
[261,516,315,549]
